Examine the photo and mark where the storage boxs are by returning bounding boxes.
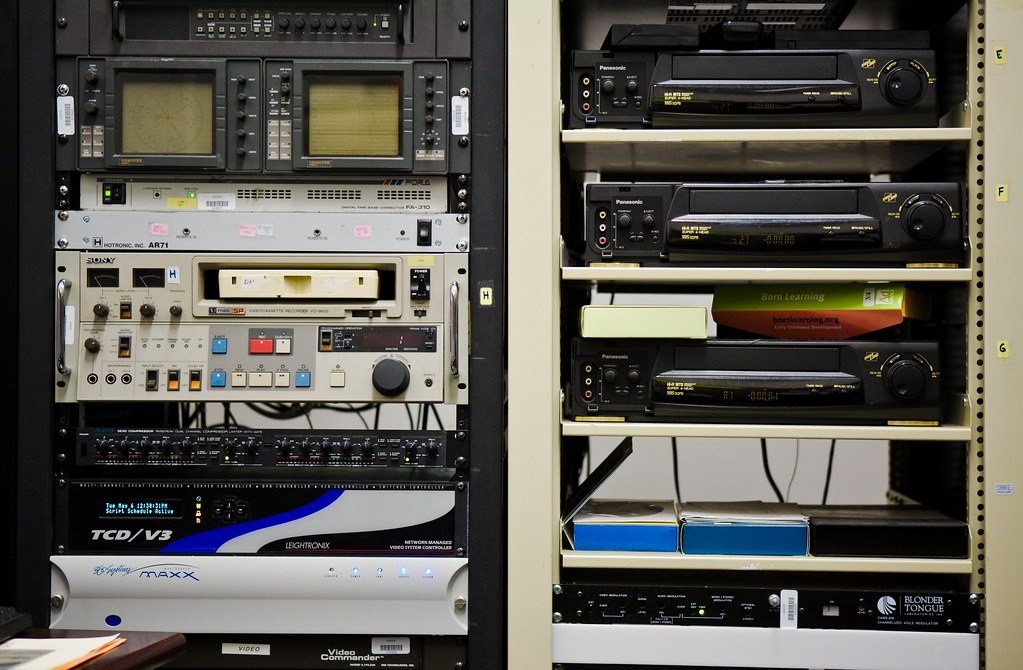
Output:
[577,304,707,339]
[573,500,679,551]
[677,503,810,555]
[801,505,968,558]
[711,284,936,343]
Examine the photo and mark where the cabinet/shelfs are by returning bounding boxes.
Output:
[504,0,1023,670]
